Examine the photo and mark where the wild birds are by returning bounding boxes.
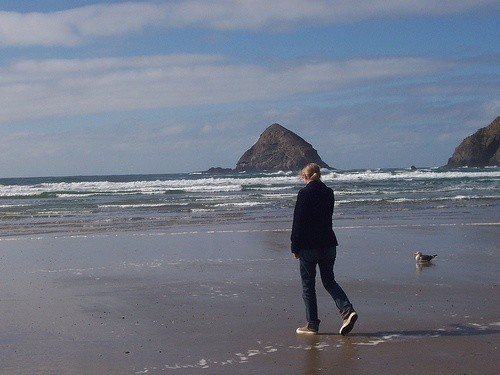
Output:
[414,251,437,262]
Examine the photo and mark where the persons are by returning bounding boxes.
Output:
[290,163,358,336]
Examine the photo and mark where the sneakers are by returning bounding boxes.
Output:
[295,326,318,335]
[338,311,358,336]
